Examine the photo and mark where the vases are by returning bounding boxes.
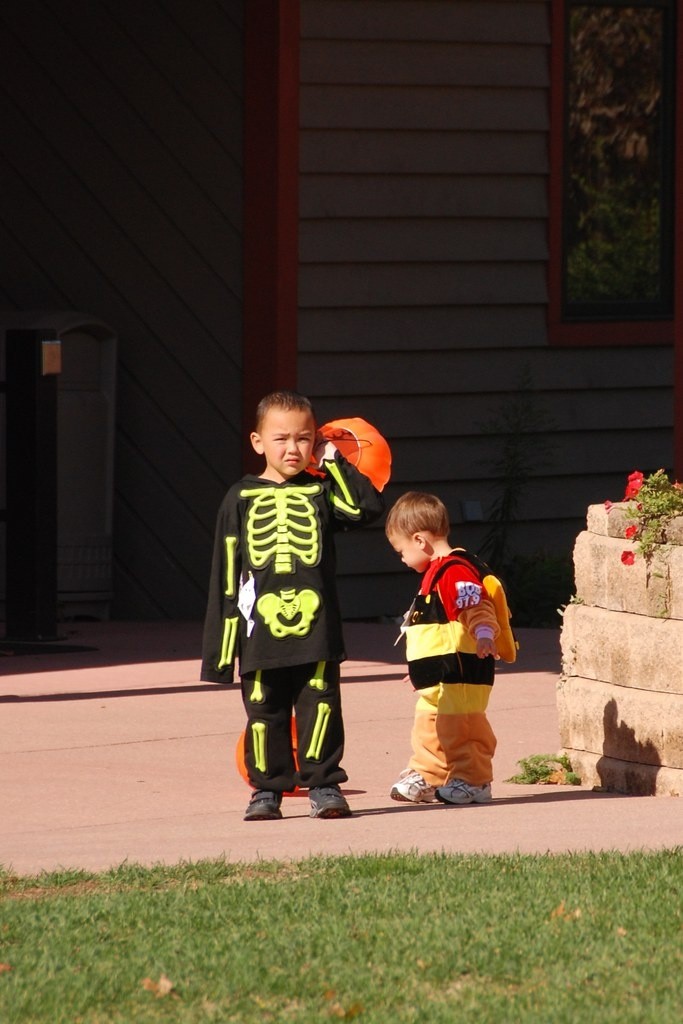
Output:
[555,501,683,798]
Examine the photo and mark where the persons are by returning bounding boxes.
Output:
[201,391,388,821]
[386,491,500,806]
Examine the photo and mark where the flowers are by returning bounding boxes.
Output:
[601,464,683,623]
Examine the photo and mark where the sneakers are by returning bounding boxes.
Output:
[436,779,493,803]
[391,769,438,803]
[309,784,351,819]
[243,788,282,819]
